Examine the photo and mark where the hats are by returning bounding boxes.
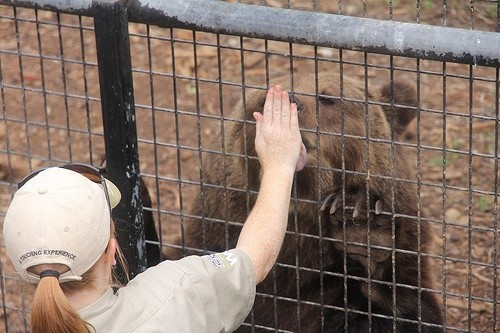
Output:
[3,167,122,284]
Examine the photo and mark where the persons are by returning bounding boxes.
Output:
[2,85,304,333]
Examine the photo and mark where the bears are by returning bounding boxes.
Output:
[163,70,445,333]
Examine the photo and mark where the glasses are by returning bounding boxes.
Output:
[18,163,112,214]
